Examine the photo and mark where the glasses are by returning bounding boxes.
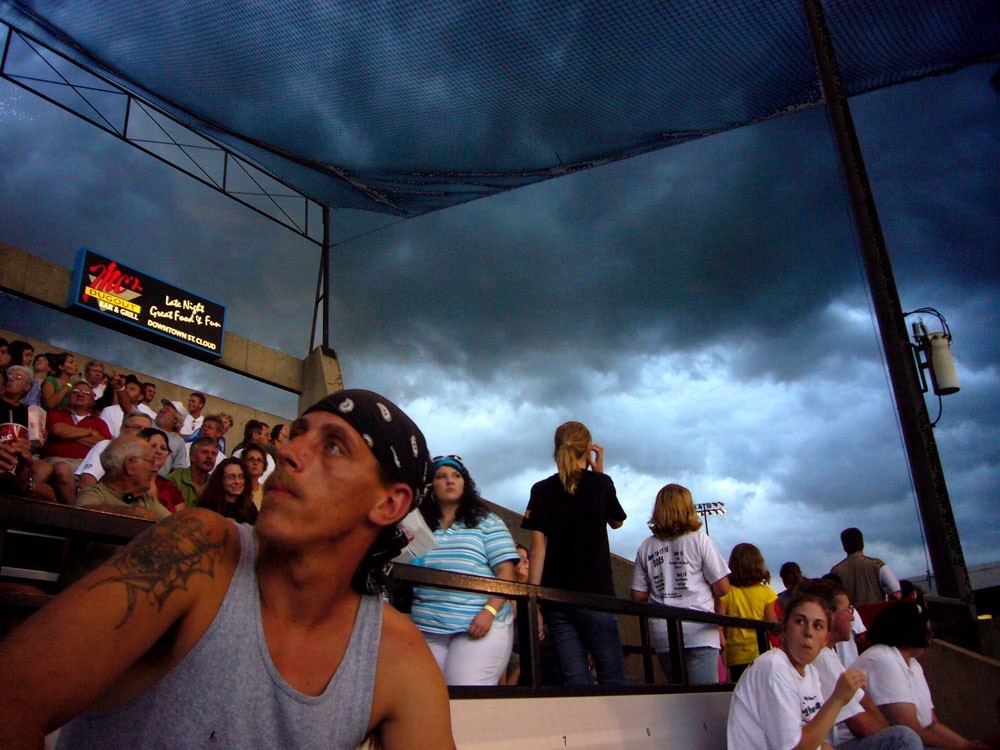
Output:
[131,456,156,465]
[73,389,91,396]
[124,424,141,432]
[433,454,462,461]
[9,374,29,385]
[838,605,854,614]
[246,457,262,462]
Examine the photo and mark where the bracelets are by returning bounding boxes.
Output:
[114,386,125,391]
[89,427,92,436]
[66,383,72,389]
[484,604,497,617]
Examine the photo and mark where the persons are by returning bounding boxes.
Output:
[500,544,545,685]
[632,484,733,684]
[0,389,456,750]
[520,422,628,687]
[408,456,521,685]
[716,525,975,750]
[0,338,289,596]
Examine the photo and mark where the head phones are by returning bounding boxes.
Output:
[109,486,136,504]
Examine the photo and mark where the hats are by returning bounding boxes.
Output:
[161,398,186,419]
[125,374,145,396]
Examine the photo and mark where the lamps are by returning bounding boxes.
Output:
[910,321,960,395]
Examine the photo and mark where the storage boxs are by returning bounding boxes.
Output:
[27,405,46,447]
